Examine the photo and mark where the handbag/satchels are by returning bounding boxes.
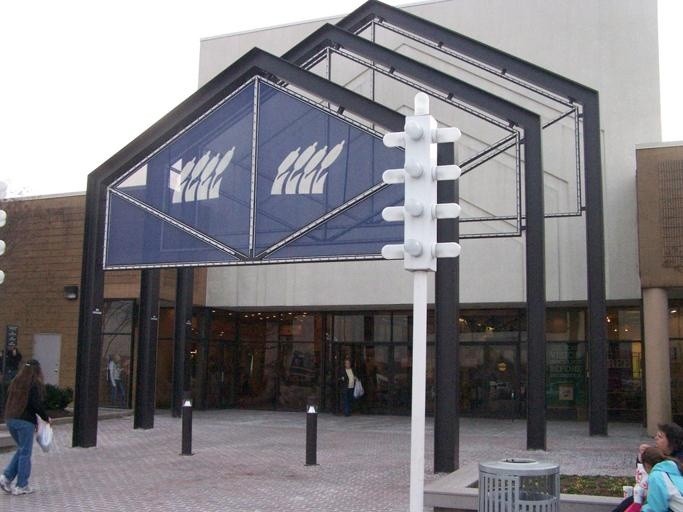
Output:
[354,380,364,398]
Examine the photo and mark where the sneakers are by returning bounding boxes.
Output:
[0,475,12,493]
[14,485,34,496]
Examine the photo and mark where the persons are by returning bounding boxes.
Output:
[0,359,54,496]
[107,353,126,410]
[622,446,682,512]
[339,358,358,417]
[5,344,22,379]
[608,421,682,512]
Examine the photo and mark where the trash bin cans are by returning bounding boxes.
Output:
[479,456,560,512]
